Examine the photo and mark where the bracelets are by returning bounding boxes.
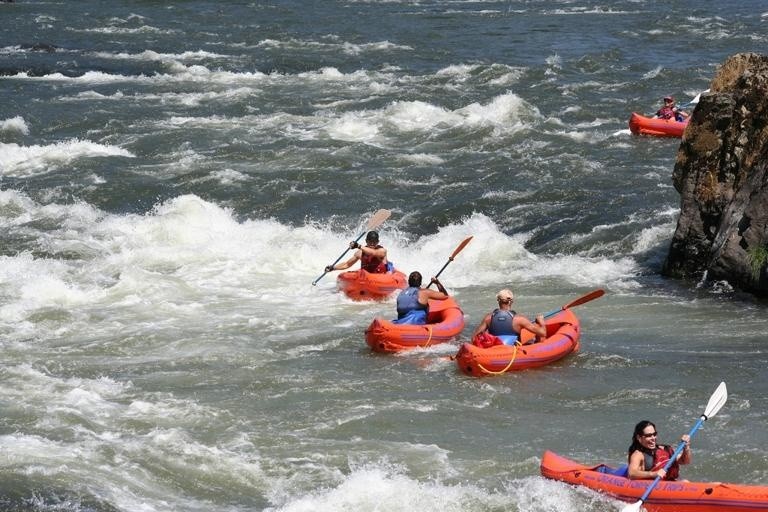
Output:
[358,244,362,250]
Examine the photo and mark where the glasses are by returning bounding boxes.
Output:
[643,432,657,437]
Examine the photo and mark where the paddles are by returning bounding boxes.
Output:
[659,88,711,118]
[311,209,391,286]
[624,381,728,512]
[426,236,473,288]
[530,288,605,322]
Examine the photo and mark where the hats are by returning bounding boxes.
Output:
[496,289,513,302]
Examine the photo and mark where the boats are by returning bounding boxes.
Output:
[451,303,582,378]
[535,450,767,511]
[627,108,693,138]
[363,289,469,357]
[333,265,411,305]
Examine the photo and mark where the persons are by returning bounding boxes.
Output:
[626,420,693,484]
[324,230,388,274]
[397,271,449,325]
[651,95,689,122]
[472,288,547,347]
